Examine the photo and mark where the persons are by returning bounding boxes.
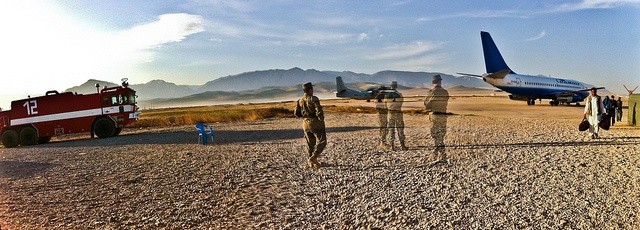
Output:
[294,81,327,168]
[384,80,409,150]
[584,87,605,139]
[374,85,388,148]
[603,95,611,116]
[424,74,449,161]
[610,95,617,126]
[617,97,623,121]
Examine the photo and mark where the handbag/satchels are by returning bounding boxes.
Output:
[600,113,610,130]
[579,117,590,131]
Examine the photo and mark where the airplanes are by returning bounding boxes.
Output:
[336,77,400,102]
[456,31,605,106]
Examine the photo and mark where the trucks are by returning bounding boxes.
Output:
[0,78,139,147]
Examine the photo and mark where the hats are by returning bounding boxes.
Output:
[302,82,315,90]
[391,80,397,86]
[432,75,442,81]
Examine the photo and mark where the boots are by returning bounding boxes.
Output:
[309,154,321,168]
[399,141,408,150]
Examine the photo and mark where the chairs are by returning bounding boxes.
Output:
[194,123,214,144]
